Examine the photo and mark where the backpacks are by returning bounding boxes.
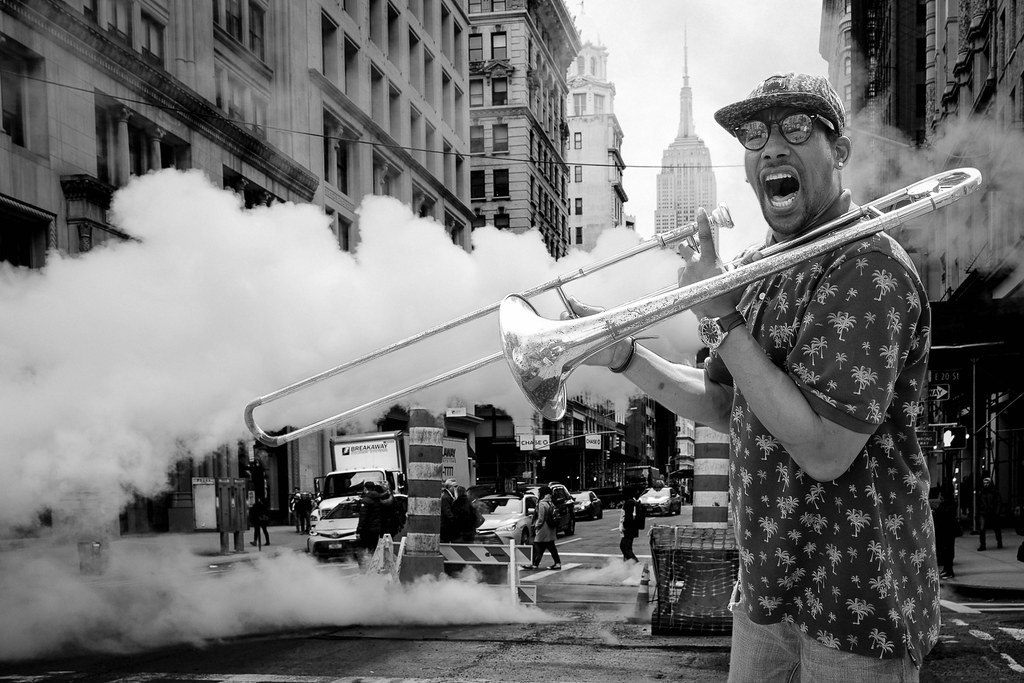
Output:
[626,499,646,530]
[544,501,560,528]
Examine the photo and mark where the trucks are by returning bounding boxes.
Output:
[309,429,472,535]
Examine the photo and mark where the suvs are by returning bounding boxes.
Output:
[512,481,576,539]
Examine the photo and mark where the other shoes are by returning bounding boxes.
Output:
[249,540,256,546]
[296,528,310,535]
[263,541,270,546]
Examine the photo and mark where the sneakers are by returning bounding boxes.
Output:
[996,542,1003,548]
[521,564,538,570]
[939,569,954,580]
[546,562,561,569]
[977,544,986,551]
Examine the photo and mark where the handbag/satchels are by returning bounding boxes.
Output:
[473,508,486,529]
[954,518,964,538]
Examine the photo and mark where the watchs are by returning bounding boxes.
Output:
[697,311,746,351]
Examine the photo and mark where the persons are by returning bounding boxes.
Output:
[440,477,465,543]
[455,486,479,545]
[928,486,956,578]
[618,486,639,564]
[373,483,403,541]
[524,485,563,571]
[977,478,1004,551]
[250,484,317,546]
[357,482,383,559]
[552,70,944,683]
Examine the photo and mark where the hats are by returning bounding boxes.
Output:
[714,73,846,137]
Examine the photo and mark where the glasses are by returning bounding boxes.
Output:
[732,111,836,152]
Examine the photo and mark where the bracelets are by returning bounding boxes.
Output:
[608,335,663,376]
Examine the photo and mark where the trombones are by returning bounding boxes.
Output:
[244,167,982,447]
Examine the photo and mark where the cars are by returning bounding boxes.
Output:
[305,498,370,568]
[637,486,682,517]
[469,491,542,547]
[568,490,603,521]
[465,484,496,503]
